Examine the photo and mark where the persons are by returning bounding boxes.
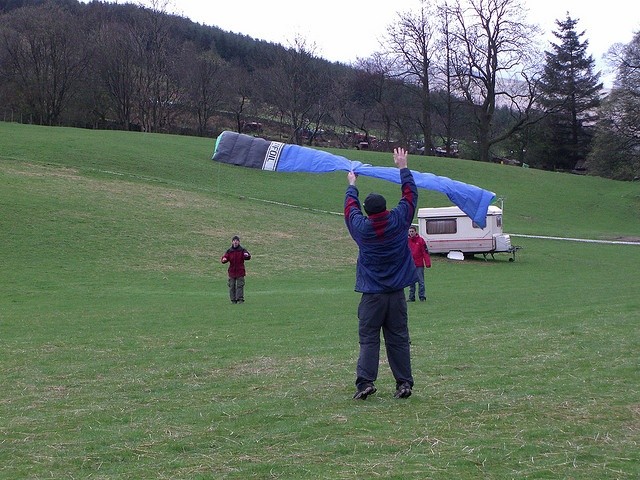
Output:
[405,226,431,303]
[221,236,251,305]
[344,147,421,399]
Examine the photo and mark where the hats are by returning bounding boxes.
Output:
[362,192,386,212]
[231,235,239,241]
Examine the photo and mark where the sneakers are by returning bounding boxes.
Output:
[393,382,411,398]
[352,382,376,399]
